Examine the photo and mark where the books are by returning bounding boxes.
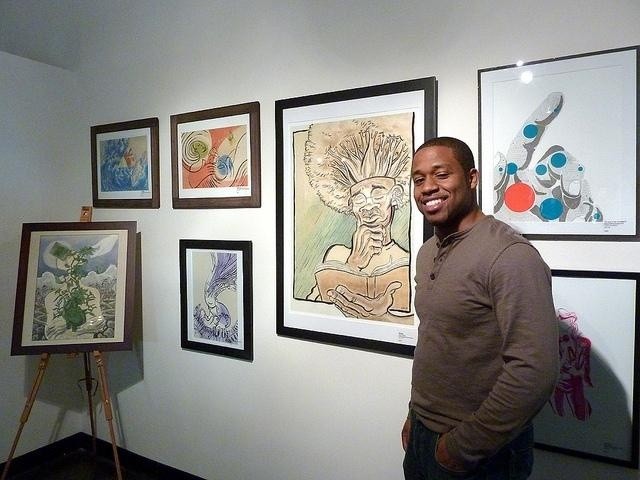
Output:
[313,256,410,313]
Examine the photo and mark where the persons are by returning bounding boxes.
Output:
[401,136,560,479]
[493,92,604,223]
[302,113,417,326]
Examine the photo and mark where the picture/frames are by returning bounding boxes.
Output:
[169,101,262,209]
[275,76,438,359]
[90,117,161,209]
[11,220,137,356]
[534,270,640,468]
[178,239,253,362]
[478,45,640,242]
